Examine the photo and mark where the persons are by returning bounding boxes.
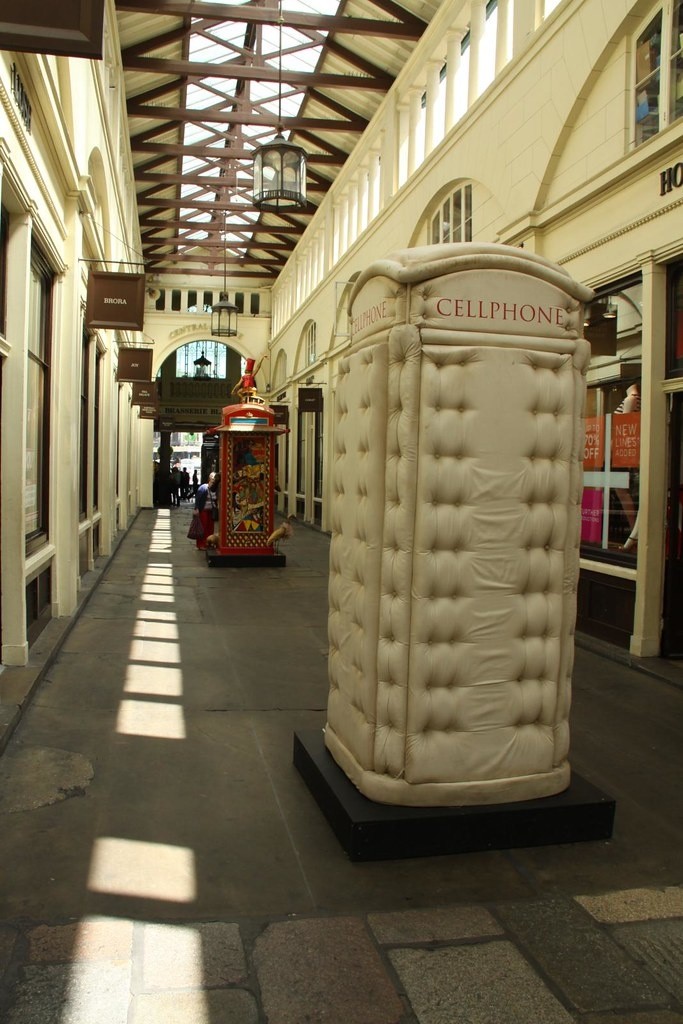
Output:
[231,356,268,402]
[169,466,182,507]
[180,467,190,500]
[187,470,198,501]
[188,473,220,551]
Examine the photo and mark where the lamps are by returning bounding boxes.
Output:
[249,0,309,211]
[211,208,239,337]
[193,351,212,381]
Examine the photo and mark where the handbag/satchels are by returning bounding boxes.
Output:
[212,507,219,521]
[187,514,211,540]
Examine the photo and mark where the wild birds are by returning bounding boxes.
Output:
[265,513,297,554]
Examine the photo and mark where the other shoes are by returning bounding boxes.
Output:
[199,547,207,551]
